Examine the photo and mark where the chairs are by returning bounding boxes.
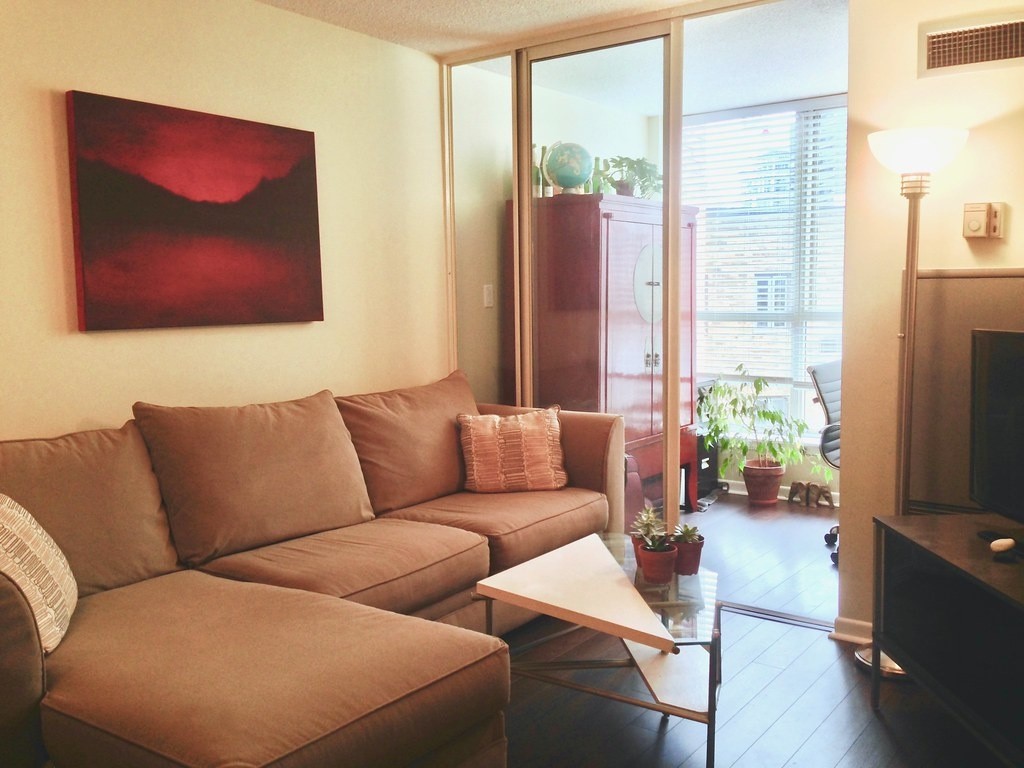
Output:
[805,360,843,567]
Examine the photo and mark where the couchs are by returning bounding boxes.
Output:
[0,371,626,768]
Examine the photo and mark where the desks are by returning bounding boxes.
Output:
[476,530,723,768]
[870,513,1024,768]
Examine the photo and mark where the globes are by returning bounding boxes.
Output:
[542,141,594,195]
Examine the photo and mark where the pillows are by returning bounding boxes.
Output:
[0,418,189,599]
[333,370,481,519]
[132,389,377,568]
[0,491,79,655]
[453,404,570,492]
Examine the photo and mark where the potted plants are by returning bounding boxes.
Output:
[601,155,664,200]
[628,504,705,584]
[695,363,833,508]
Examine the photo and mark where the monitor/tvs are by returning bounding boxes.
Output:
[970,329,1024,558]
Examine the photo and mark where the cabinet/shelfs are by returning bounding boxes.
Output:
[499,198,700,535]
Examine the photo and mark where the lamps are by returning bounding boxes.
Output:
[854,124,950,684]
